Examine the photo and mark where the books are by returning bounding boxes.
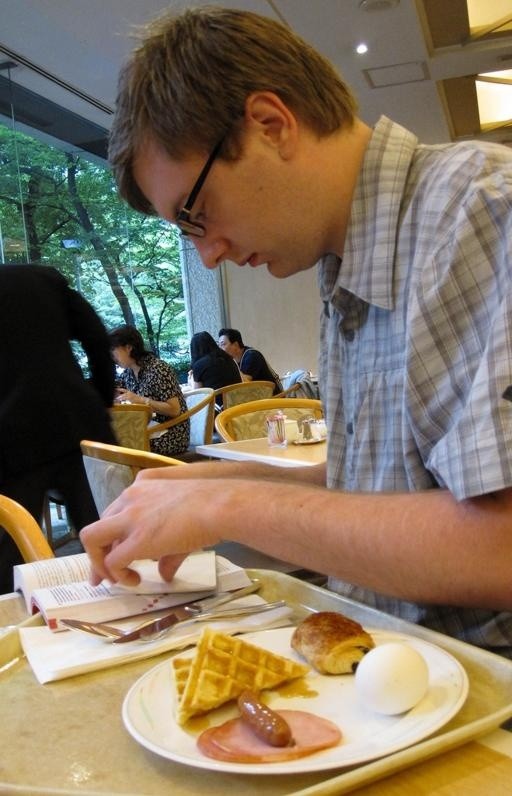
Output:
[12,540,254,633]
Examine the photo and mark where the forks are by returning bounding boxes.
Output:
[114,583,264,645]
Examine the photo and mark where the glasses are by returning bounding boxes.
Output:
[178,138,223,240]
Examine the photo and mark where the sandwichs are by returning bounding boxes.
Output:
[177,626,306,724]
[171,657,200,701]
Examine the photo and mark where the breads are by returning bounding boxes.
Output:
[290,610,375,675]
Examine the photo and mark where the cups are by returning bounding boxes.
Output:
[266,414,287,449]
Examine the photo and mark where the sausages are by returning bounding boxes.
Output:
[237,688,291,743]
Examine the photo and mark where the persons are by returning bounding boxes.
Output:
[105,325,190,456]
[77,8,511,660]
[188,329,245,433]
[0,262,127,593]
[214,326,284,398]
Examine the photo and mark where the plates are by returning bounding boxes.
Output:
[292,438,327,446]
[121,624,470,777]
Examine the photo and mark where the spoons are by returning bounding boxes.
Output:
[53,598,289,643]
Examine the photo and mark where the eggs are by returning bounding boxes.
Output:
[355,641,430,715]
